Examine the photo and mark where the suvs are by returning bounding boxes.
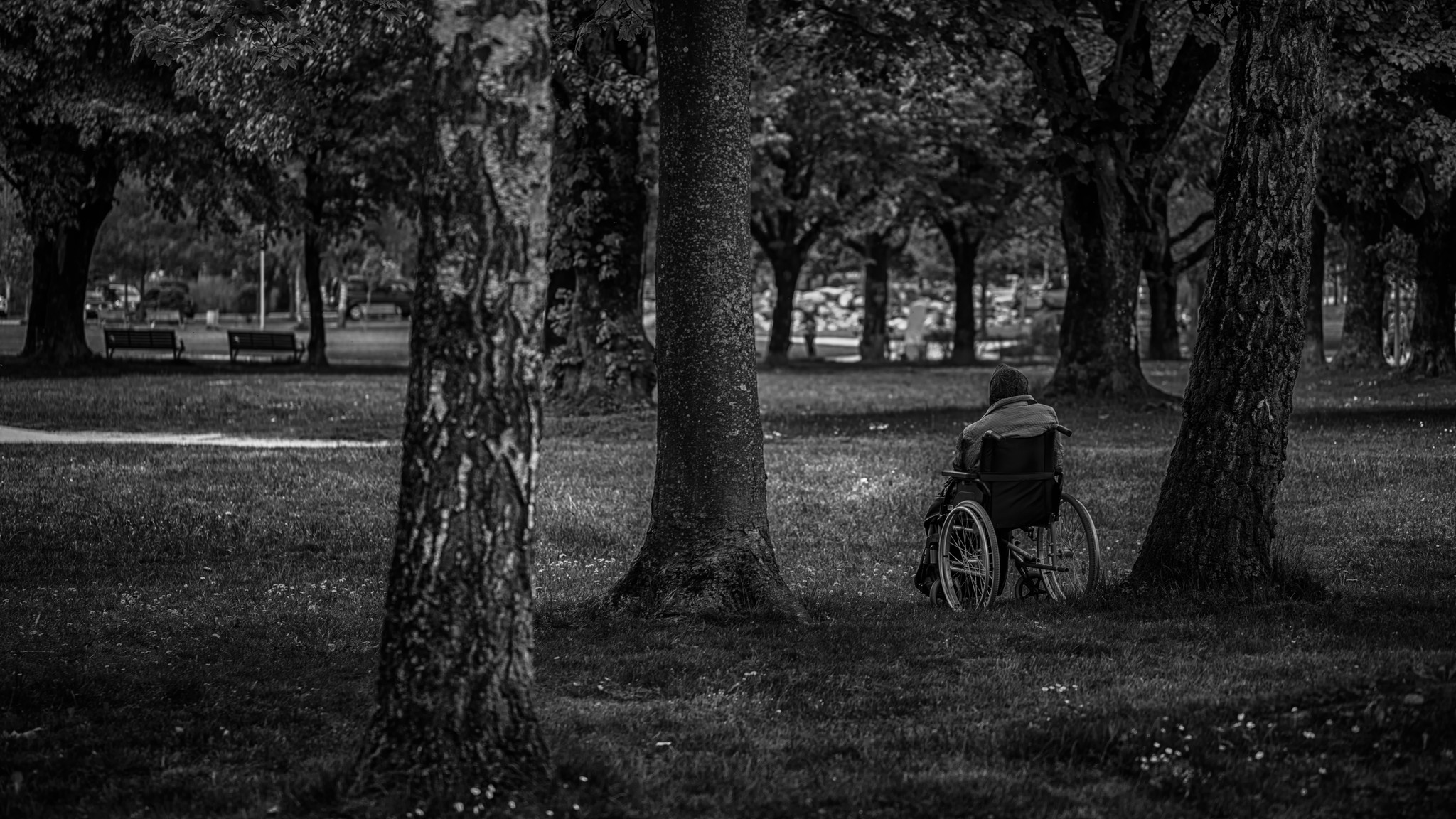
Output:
[327,273,414,322]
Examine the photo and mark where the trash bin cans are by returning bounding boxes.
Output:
[205,308,218,329]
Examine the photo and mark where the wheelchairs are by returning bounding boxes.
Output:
[916,423,1100,617]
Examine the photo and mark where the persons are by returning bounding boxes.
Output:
[803,312,817,357]
[924,363,1065,585]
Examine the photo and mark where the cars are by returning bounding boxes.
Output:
[754,269,1068,350]
[83,274,199,326]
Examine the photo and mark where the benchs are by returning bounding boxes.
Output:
[360,305,402,321]
[102,328,184,360]
[97,311,130,328]
[146,308,185,328]
[227,330,305,365]
